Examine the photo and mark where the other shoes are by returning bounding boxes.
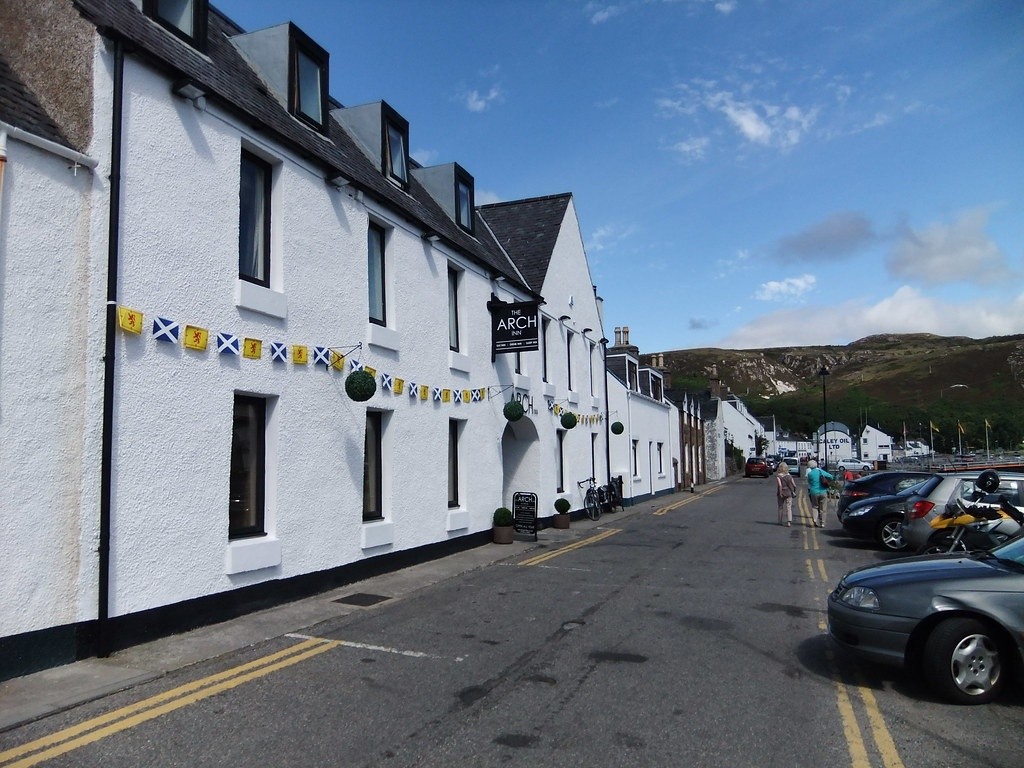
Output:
[786,523,791,527]
[813,520,818,527]
[821,524,825,528]
[778,523,783,526]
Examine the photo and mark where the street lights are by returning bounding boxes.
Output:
[819,364,831,473]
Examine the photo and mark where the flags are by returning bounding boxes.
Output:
[985,418,992,429]
[930,421,940,433]
[903,421,911,436]
[958,420,964,434]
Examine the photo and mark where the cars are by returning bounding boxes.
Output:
[744,453,802,479]
[836,469,1024,552]
[823,528,1024,706]
[835,458,875,471]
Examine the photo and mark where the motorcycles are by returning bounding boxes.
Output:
[915,491,1024,555]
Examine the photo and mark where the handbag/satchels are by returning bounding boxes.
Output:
[819,470,829,488]
[792,491,796,498]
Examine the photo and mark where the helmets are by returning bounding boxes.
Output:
[976,470,1000,493]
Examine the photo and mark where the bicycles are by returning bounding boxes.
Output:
[577,476,618,521]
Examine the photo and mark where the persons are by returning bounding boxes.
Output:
[834,471,840,483]
[844,468,853,485]
[806,460,833,528]
[855,471,863,479]
[776,462,797,527]
